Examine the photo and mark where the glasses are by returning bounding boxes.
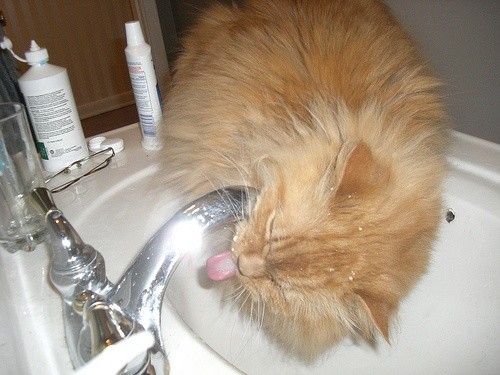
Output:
[43,147,115,194]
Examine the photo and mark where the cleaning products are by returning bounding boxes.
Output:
[0,36,90,172]
[125,20,164,153]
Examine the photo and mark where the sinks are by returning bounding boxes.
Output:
[27,149,500,375]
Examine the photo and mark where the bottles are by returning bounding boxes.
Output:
[123,20,166,149]
[0,36,88,172]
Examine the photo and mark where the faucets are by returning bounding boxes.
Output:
[76,184,259,375]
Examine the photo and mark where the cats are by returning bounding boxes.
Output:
[152,1,462,368]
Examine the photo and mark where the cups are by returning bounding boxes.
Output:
[0,101,57,254]
